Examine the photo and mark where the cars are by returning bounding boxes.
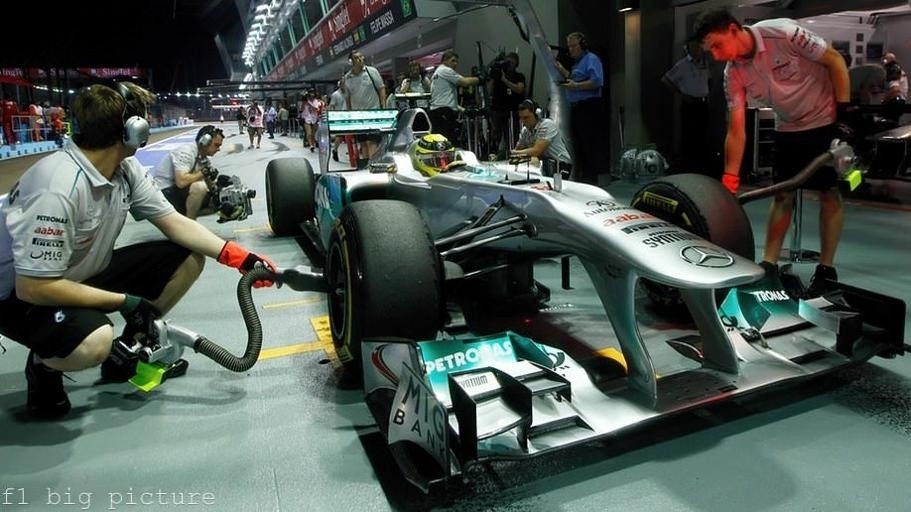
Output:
[266,93,911,500]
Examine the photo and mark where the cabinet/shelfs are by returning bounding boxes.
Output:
[746,107,775,176]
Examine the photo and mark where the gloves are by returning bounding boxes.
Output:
[216,241,282,289]
[118,293,162,332]
[201,166,218,181]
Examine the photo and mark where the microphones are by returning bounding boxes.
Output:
[523,124,525,127]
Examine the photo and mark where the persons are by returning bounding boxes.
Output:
[384,60,430,112]
[510,100,573,182]
[834,52,910,184]
[151,125,256,222]
[693,5,850,296]
[426,51,483,149]
[486,51,526,152]
[0,97,66,147]
[554,31,605,183]
[235,98,292,150]
[661,36,716,176]
[297,88,332,153]
[0,81,277,424]
[340,50,387,165]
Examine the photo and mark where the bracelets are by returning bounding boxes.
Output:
[575,81,579,92]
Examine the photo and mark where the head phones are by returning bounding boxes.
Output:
[348,54,365,66]
[577,32,588,48]
[90,79,148,149]
[198,127,218,146]
[523,99,543,120]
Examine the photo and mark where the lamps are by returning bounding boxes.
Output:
[616,0,633,13]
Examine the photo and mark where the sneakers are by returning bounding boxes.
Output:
[807,264,838,297]
[24,350,71,417]
[101,337,189,384]
